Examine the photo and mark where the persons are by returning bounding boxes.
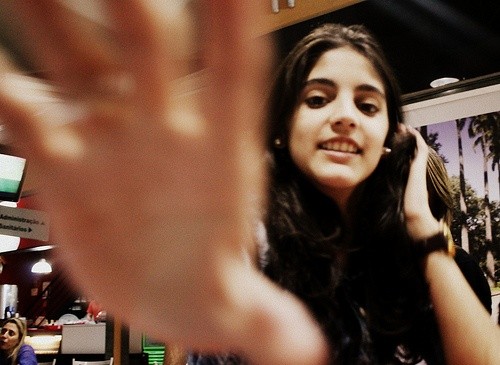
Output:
[84,292,109,327]
[0,298,37,365]
[0,1,500,365]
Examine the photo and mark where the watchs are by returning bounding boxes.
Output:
[404,215,456,260]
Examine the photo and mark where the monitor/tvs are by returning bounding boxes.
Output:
[0,144,28,203]
